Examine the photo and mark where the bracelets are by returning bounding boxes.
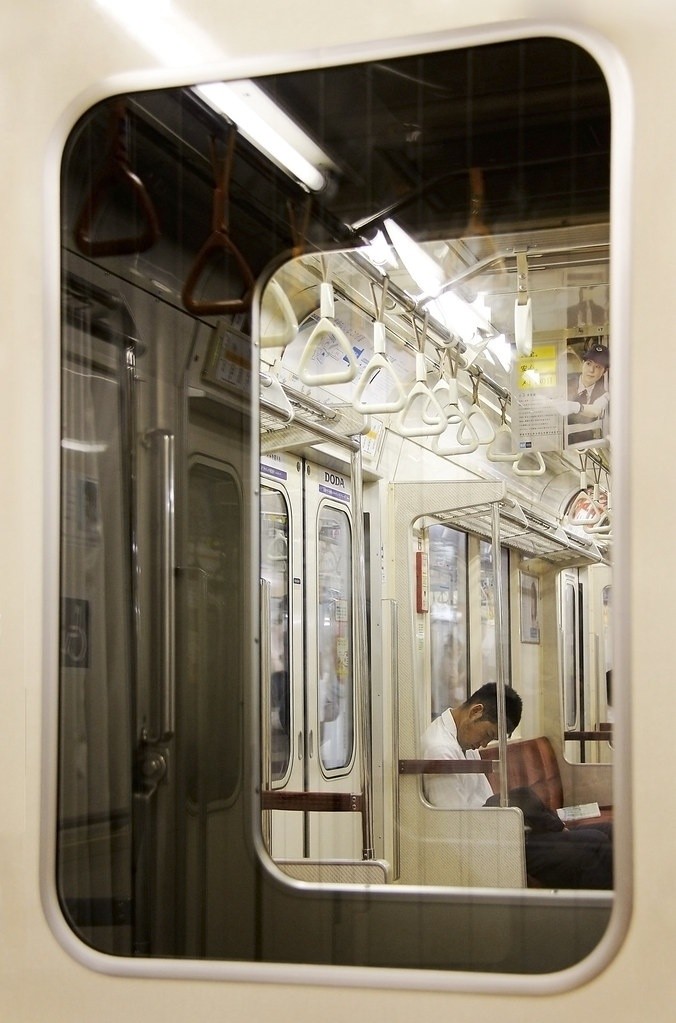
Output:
[575,401,583,416]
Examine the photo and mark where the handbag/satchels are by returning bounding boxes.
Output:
[483,785,565,832]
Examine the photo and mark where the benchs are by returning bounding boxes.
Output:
[397,733,615,891]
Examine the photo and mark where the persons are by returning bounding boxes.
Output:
[556,344,609,445]
[270,597,340,727]
[419,682,613,889]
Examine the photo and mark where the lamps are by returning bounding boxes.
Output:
[183,76,369,211]
